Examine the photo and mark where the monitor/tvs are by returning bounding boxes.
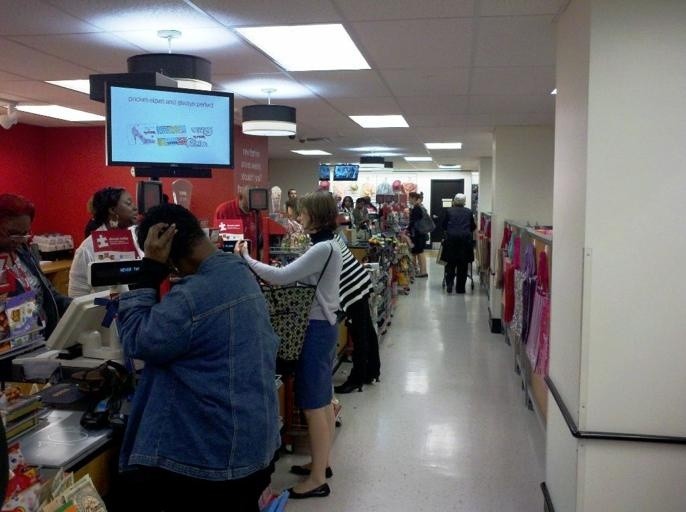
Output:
[334,164,359,181]
[46,289,111,349]
[105,81,235,169]
[319,165,330,181]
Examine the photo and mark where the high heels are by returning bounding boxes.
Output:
[333,368,381,393]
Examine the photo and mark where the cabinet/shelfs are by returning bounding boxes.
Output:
[39,260,74,298]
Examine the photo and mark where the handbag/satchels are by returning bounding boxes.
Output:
[495,224,550,380]
[437,244,447,267]
[413,215,437,233]
[250,279,314,363]
[475,214,491,276]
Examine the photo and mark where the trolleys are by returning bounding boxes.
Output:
[441,238,477,291]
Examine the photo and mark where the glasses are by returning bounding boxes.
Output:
[1,225,35,242]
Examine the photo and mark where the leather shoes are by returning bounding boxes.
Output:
[289,459,334,477]
[282,482,331,499]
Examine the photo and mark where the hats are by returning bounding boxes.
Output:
[392,178,418,192]
[319,181,331,191]
[350,183,359,193]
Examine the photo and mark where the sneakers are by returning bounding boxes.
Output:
[415,270,466,294]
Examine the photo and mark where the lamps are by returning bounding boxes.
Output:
[360,151,393,170]
[126,30,212,91]
[241,89,297,136]
[0,100,18,129]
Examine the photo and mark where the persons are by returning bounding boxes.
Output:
[1,185,473,512]
